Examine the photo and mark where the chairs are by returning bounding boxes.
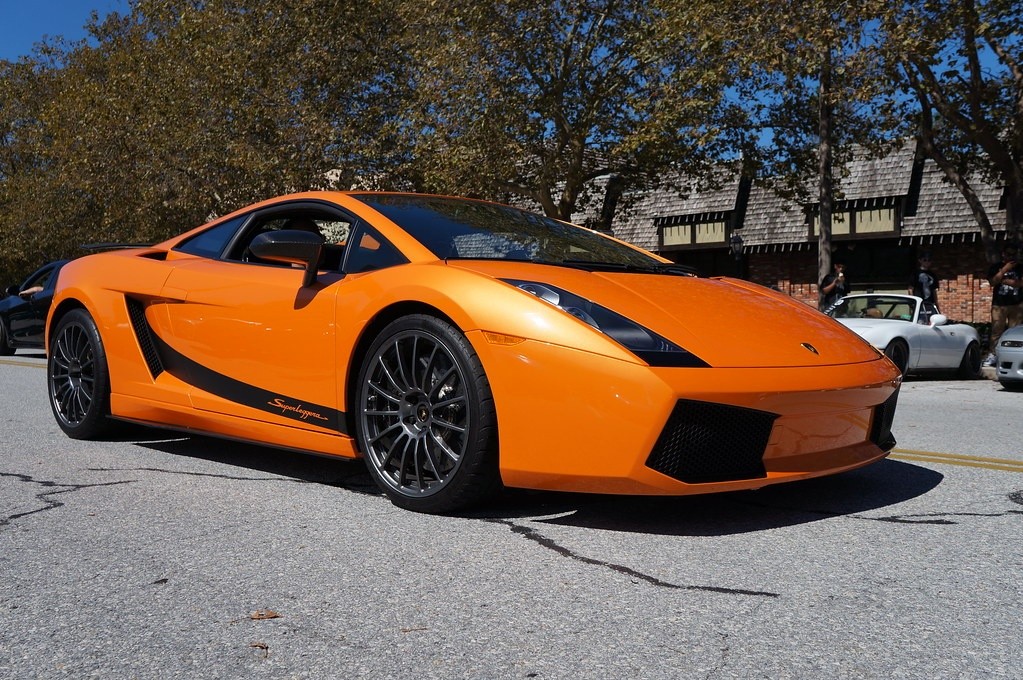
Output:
[866,308,883,319]
[281,217,328,271]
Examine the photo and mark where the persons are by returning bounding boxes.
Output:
[989,243,1023,355]
[819,258,851,318]
[907,252,941,322]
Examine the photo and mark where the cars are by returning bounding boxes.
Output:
[0,255,71,357]
[993,324,1023,389]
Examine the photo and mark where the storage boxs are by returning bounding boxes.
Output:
[935,273,994,323]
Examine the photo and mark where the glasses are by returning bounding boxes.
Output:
[923,259,931,262]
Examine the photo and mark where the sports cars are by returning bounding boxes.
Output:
[823,291,983,381]
[43,190,905,529]
